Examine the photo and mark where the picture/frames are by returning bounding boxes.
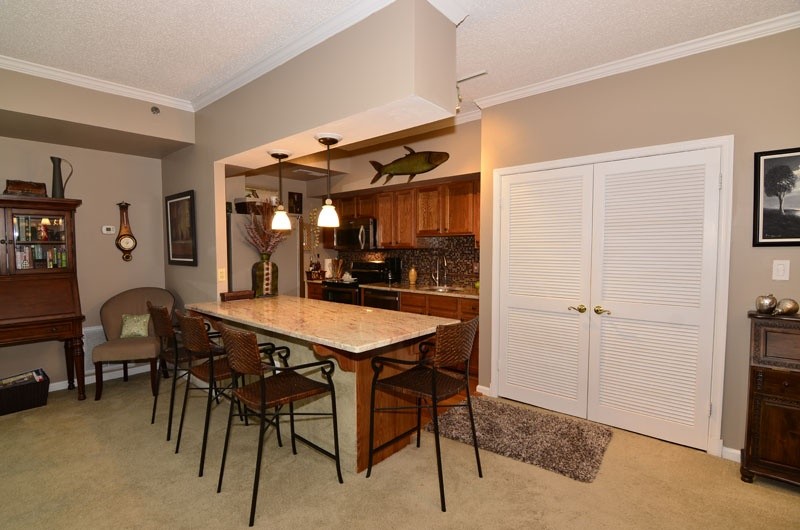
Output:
[164,189,198,267]
[752,147,800,248]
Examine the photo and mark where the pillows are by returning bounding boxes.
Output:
[120,313,149,338]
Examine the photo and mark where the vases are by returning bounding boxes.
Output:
[251,253,280,297]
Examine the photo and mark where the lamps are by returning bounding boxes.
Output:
[269,153,293,231]
[314,133,341,229]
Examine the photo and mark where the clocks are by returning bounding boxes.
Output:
[116,201,138,262]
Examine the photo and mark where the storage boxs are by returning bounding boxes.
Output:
[0,368,50,416]
[234,196,278,215]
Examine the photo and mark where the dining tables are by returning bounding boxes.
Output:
[191,292,461,474]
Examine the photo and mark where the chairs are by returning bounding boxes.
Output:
[144,301,224,441]
[216,323,345,526]
[90,287,176,398]
[364,318,484,512]
[173,309,281,477]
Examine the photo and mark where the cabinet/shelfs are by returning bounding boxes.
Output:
[459,297,479,378]
[473,179,480,250]
[739,311,800,491]
[321,198,342,248]
[415,184,474,236]
[339,194,376,220]
[307,283,320,300]
[399,293,459,324]
[0,194,88,402]
[377,192,434,251]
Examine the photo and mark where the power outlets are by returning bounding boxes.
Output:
[771,258,790,281]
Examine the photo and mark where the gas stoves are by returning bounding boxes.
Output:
[322,260,384,289]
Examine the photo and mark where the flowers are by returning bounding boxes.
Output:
[237,207,296,254]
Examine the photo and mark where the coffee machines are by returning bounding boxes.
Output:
[384,258,402,284]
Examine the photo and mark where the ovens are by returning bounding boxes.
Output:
[323,286,360,307]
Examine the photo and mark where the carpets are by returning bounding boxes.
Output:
[426,395,605,488]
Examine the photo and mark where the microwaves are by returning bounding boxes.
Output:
[334,218,378,252]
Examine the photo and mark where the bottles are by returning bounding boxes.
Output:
[309,256,314,270]
[53,246,66,268]
[315,253,322,272]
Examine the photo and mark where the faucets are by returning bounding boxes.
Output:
[436,254,447,292]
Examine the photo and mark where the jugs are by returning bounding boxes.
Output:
[50,155,73,198]
